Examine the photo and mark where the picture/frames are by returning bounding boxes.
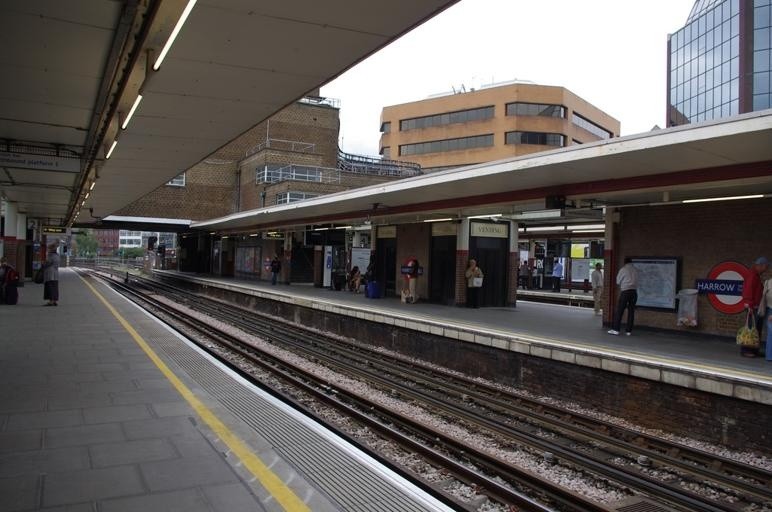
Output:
[623,253,684,314]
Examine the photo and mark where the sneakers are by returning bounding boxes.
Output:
[608,329,619,335]
[743,351,755,357]
[624,331,631,336]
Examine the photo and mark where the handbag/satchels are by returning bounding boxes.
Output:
[473,277,483,287]
[757,295,767,316]
[736,327,760,348]
[32,268,44,284]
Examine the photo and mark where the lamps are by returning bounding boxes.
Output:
[314,225,331,232]
[149,0,201,77]
[98,120,123,163]
[61,165,101,230]
[423,212,460,224]
[333,224,353,229]
[681,192,767,207]
[117,65,150,134]
[464,207,512,221]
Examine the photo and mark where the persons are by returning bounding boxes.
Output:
[739,256,769,357]
[271,253,281,285]
[607,256,639,335]
[406,255,420,304]
[41,241,60,306]
[366,254,381,299]
[348,265,362,294]
[591,262,603,312]
[758,275,771,361]
[519,260,531,290]
[551,257,563,292]
[465,258,484,308]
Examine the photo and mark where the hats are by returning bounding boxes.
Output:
[755,257,768,264]
